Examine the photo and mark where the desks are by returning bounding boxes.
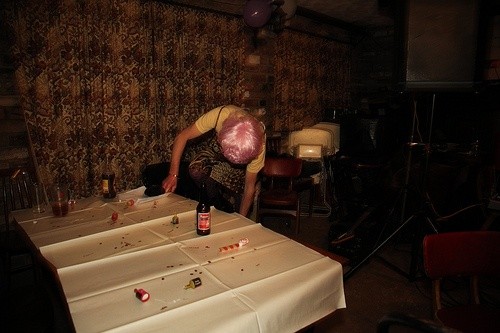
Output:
[10,186,350,333]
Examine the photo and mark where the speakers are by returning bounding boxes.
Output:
[396,0,483,92]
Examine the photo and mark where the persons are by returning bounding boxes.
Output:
[161,104,265,217]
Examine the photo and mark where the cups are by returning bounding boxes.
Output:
[31,182,45,213]
[50,182,68,215]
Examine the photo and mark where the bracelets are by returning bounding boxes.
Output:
[169,174,178,177]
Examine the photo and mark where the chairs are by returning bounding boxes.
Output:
[423,231,500,333]
[259,158,303,236]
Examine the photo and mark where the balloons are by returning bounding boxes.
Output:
[272,0,296,21]
[245,0,271,28]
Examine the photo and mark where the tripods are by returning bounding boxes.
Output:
[343,92,443,286]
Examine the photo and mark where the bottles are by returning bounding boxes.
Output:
[197,185,211,235]
[102,157,116,198]
